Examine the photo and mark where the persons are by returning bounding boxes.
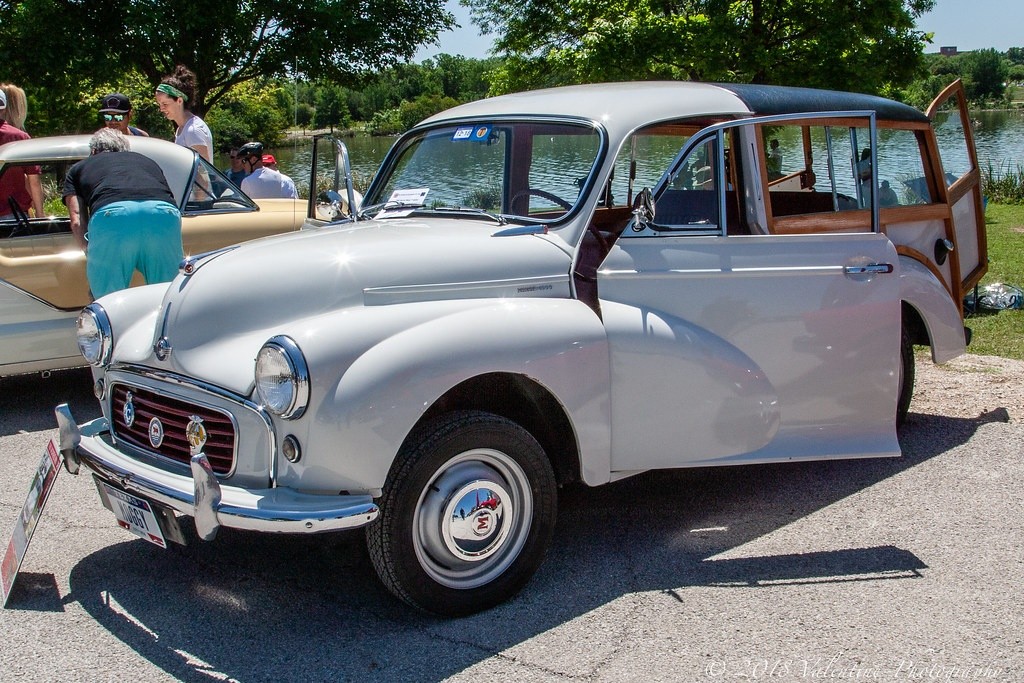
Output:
[99,93,149,136]
[62,126,184,300]
[689,152,705,172]
[156,65,214,201]
[879,179,898,207]
[859,148,871,209]
[673,160,693,190]
[0,83,46,219]
[770,139,782,173]
[222,146,251,188]
[262,154,280,172]
[236,141,299,199]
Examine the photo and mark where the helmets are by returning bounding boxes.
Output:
[237,142,263,159]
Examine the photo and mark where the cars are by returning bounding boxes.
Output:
[0,134,333,380]
[53,81,990,611]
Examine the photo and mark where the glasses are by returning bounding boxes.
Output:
[264,163,271,167]
[242,159,248,164]
[230,156,236,159]
[103,112,128,122]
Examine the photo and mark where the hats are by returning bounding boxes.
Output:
[262,155,276,163]
[0,89,7,109]
[98,93,131,113]
